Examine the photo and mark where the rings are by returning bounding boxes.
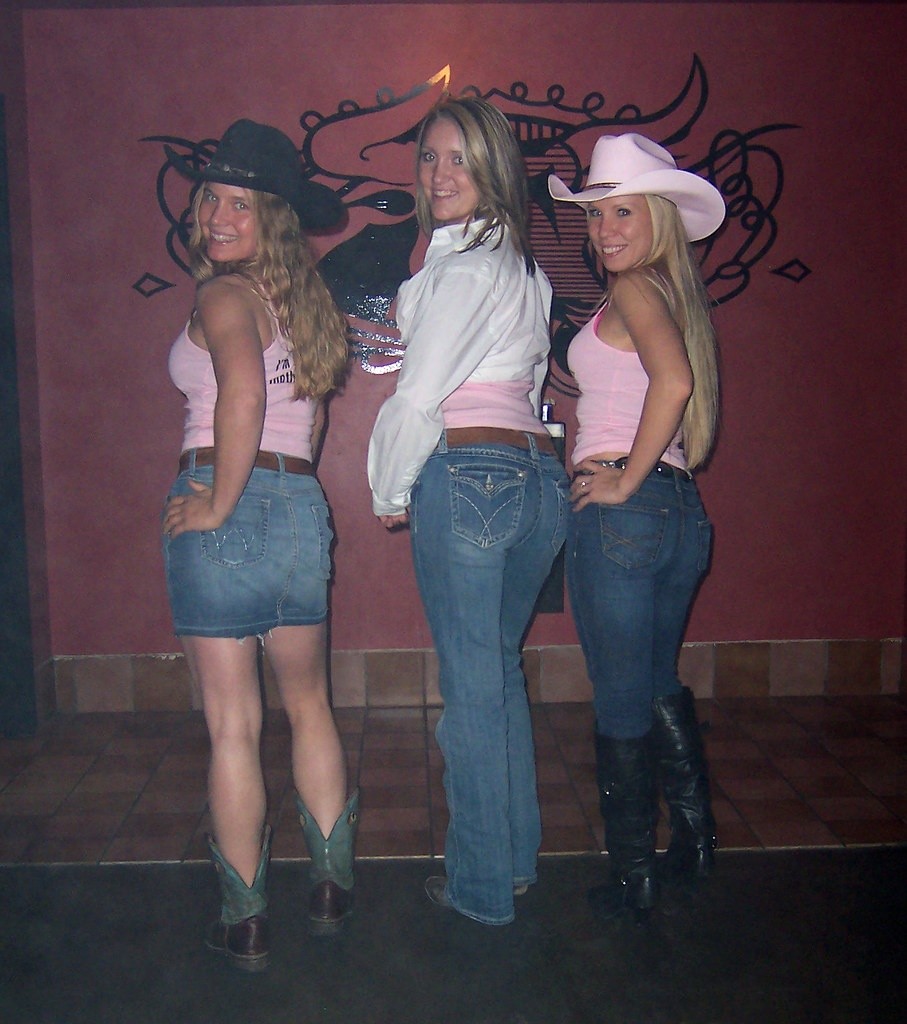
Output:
[580,481,589,496]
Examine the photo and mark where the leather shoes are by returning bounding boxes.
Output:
[425,875,454,907]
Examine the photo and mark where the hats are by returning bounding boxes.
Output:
[163,118,344,230]
[546,132,726,242]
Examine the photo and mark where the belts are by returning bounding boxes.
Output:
[570,455,691,483]
[436,427,556,454]
[177,447,316,478]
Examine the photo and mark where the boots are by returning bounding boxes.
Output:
[650,684,720,884]
[201,823,273,973]
[293,784,360,935]
[588,717,661,927]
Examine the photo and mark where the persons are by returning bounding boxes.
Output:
[544,130,723,946]
[159,119,361,973]
[362,93,576,927]
